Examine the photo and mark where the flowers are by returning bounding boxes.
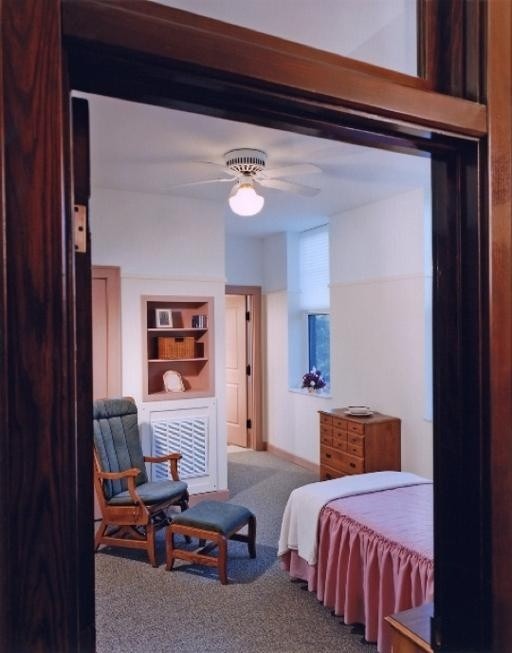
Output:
[301,365,326,389]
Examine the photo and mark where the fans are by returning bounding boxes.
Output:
[169,147,324,199]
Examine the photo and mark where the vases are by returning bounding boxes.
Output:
[308,386,323,394]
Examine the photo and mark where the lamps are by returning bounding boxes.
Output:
[228,177,265,216]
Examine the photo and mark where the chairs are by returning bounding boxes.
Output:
[93,396,194,568]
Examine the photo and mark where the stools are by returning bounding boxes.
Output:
[165,499,257,585]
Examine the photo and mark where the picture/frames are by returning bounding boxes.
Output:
[155,308,174,328]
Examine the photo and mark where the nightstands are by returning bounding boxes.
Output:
[383,603,434,652]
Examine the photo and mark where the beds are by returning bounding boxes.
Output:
[277,470,434,652]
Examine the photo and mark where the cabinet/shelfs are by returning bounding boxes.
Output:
[140,293,216,402]
[317,407,401,481]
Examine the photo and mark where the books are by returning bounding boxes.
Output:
[157,336,195,360]
